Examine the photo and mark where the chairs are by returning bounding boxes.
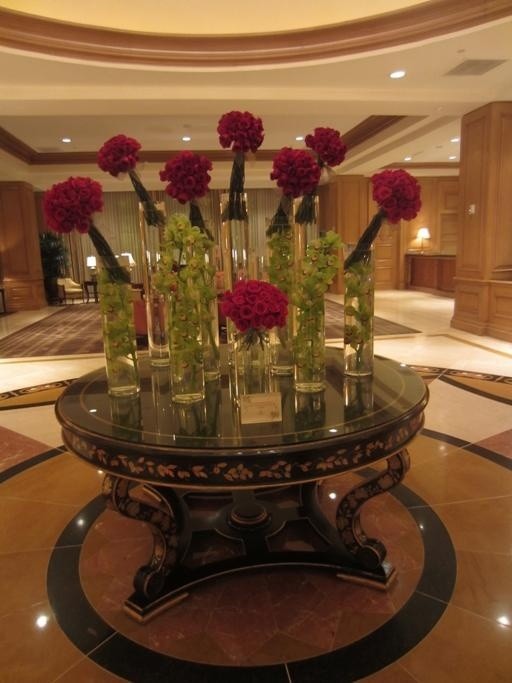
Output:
[56,276,85,304]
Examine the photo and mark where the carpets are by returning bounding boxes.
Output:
[1,290,427,361]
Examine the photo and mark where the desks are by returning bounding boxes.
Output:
[50,345,433,621]
